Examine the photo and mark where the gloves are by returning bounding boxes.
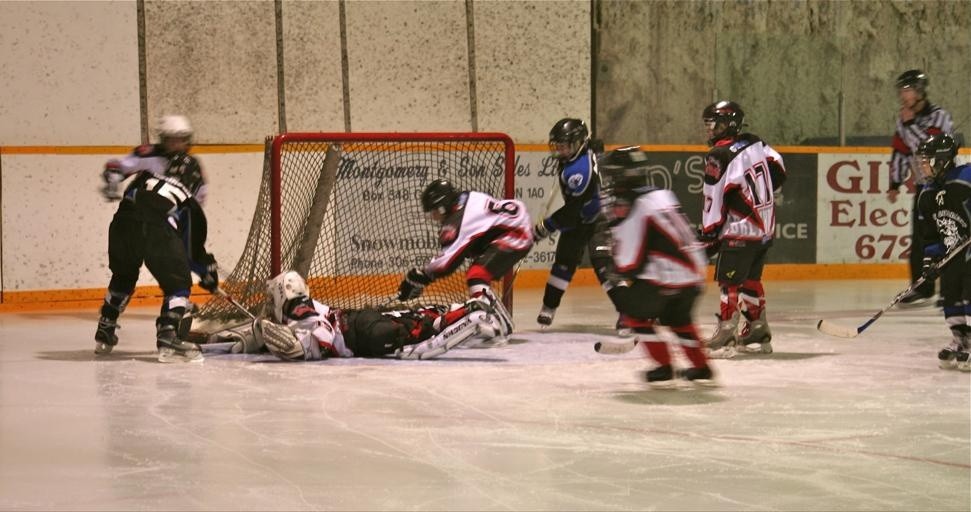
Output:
[197,253,219,293]
[398,268,431,302]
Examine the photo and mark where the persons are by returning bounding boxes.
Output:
[596,147,711,381]
[94,172,219,347]
[891,70,955,306]
[532,119,628,326]
[104,117,206,204]
[221,268,514,361]
[699,101,786,347]
[399,180,530,300]
[916,133,970,363]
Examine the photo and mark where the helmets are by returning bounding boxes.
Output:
[157,115,192,140]
[702,100,744,148]
[548,117,589,162]
[418,179,456,221]
[893,69,930,91]
[266,269,309,320]
[596,143,648,193]
[165,153,202,184]
[914,132,959,182]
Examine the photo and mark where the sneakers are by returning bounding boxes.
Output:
[675,360,712,380]
[937,329,971,364]
[638,363,673,382]
[536,305,557,325]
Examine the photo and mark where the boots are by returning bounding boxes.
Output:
[94,315,121,344]
[155,317,198,351]
[700,313,738,346]
[737,309,772,344]
